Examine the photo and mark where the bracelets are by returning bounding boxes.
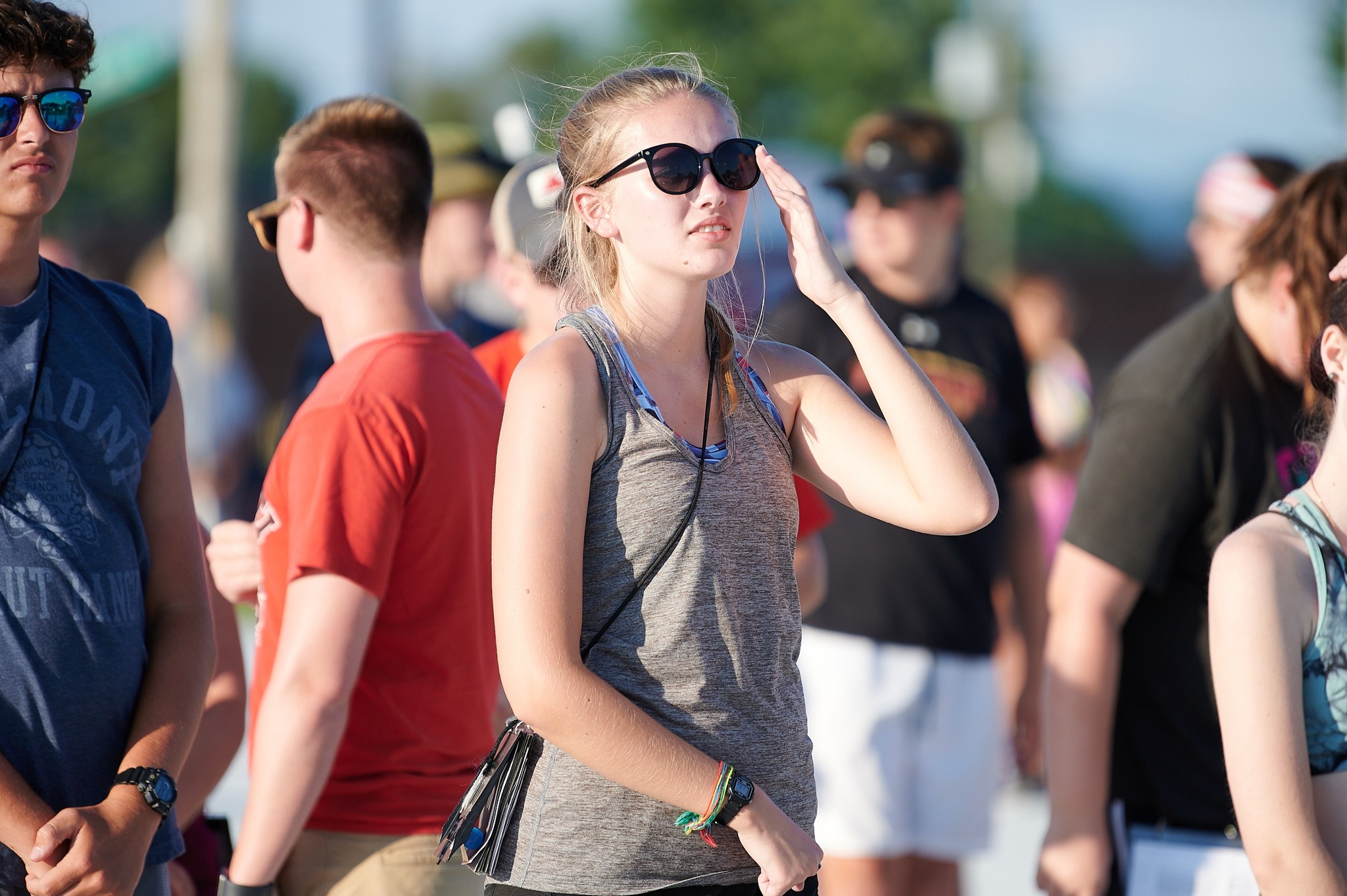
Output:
[218,874,274,896]
[676,761,734,848]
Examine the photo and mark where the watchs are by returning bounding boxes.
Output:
[114,762,180,817]
[715,770,754,827]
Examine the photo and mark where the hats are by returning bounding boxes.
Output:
[421,120,509,204]
[820,106,964,198]
[509,157,569,266]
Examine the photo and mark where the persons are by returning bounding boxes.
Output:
[1036,212,1316,896]
[1189,154,1301,287]
[1209,162,1345,896]
[204,95,526,896]
[472,147,614,404]
[748,108,1053,896]
[438,67,998,896]
[0,0,228,896]
[288,121,519,427]
[988,276,1092,793]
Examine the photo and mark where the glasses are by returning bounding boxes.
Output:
[0,88,92,139]
[246,195,321,252]
[585,137,764,233]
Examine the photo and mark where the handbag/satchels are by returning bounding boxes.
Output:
[433,723,544,878]
